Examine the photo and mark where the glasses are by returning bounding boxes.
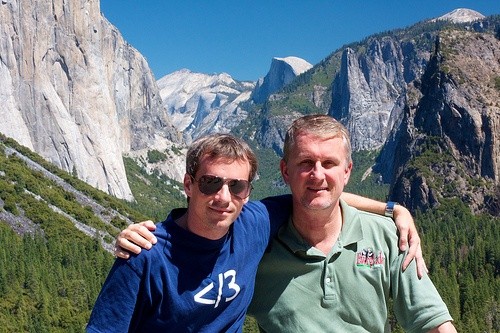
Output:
[189,174,254,200]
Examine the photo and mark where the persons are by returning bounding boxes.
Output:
[114,113,458,333]
[85,133,429,333]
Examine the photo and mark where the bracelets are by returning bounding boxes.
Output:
[385,202,400,217]
